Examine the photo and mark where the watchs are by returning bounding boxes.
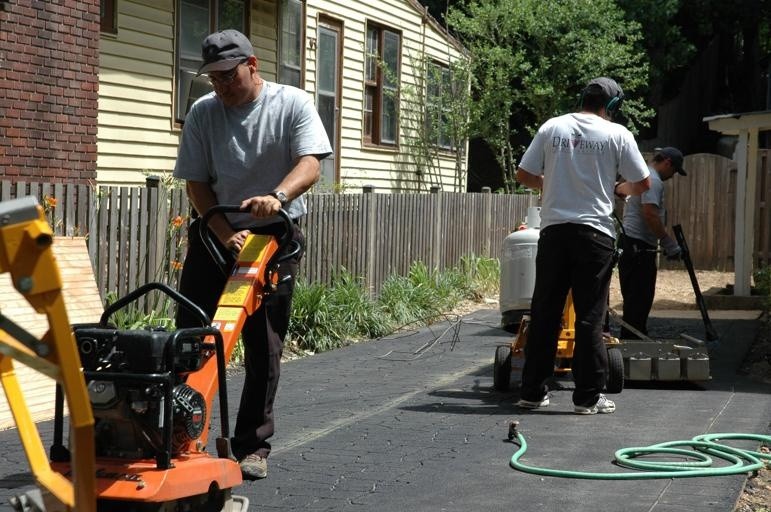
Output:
[614,180,626,200]
[269,190,290,206]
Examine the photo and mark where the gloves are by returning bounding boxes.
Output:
[660,237,680,261]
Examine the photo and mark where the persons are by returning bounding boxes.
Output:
[173,30,333,479]
[617,146,689,341]
[515,77,650,414]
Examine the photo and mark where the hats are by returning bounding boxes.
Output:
[657,147,688,175]
[583,77,625,110]
[196,29,255,77]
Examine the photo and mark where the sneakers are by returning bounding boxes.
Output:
[574,394,616,414]
[238,454,267,478]
[519,396,550,409]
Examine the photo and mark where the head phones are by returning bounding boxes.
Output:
[576,77,625,117]
